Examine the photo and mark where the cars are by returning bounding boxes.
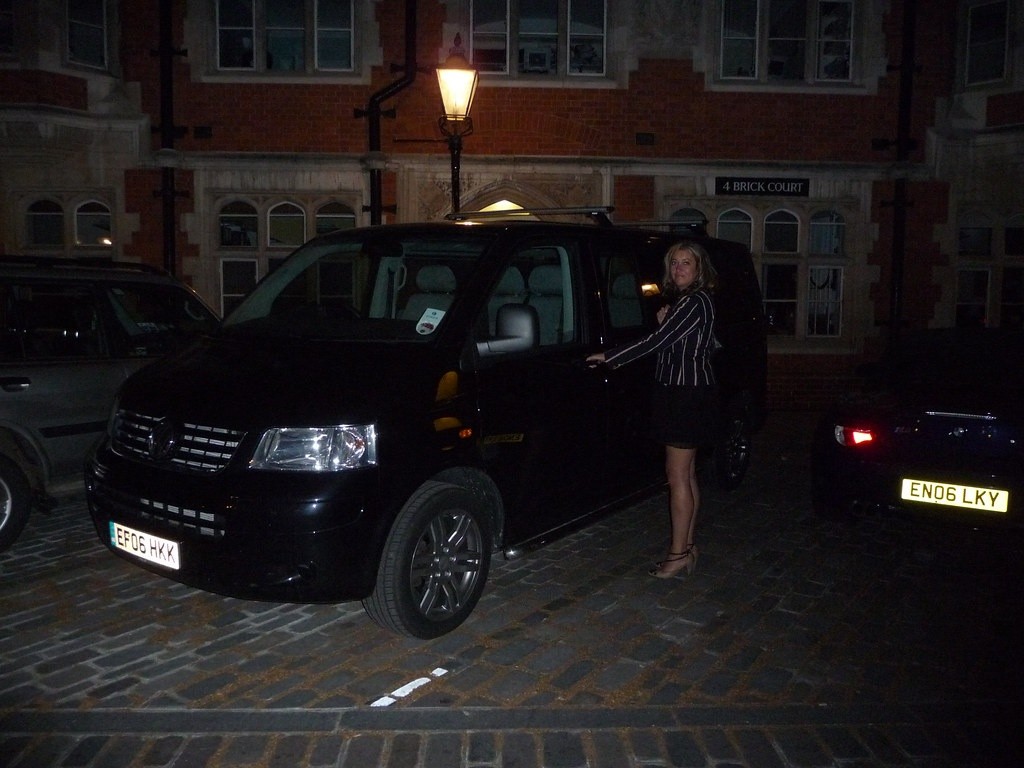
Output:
[814,328,1024,527]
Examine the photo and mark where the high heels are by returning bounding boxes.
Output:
[647,550,695,578]
[651,543,700,568]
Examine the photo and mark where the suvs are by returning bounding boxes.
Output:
[74,204,770,639]
[0,254,226,557]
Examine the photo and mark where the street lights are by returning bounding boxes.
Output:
[437,32,478,222]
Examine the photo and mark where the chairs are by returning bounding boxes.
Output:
[403,263,645,347]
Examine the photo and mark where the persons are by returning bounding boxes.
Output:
[585,240,716,579]
